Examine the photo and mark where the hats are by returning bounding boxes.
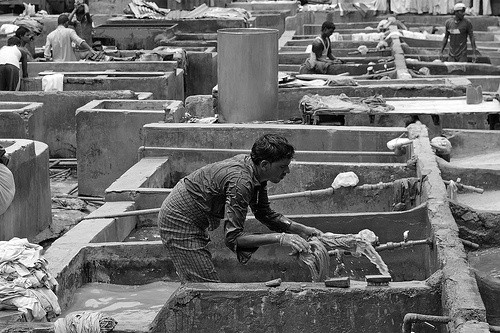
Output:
[453,2,466,10]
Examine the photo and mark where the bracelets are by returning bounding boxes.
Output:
[279,232,286,247]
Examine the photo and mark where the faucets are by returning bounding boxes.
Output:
[332,262,345,277]
[402,313,449,332]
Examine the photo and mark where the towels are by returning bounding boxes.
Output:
[42,74,64,92]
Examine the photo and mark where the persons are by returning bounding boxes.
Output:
[15,26,38,78]
[156,133,325,283]
[0,36,28,91]
[68,4,93,49]
[44,14,98,62]
[310,21,336,75]
[439,3,477,62]
